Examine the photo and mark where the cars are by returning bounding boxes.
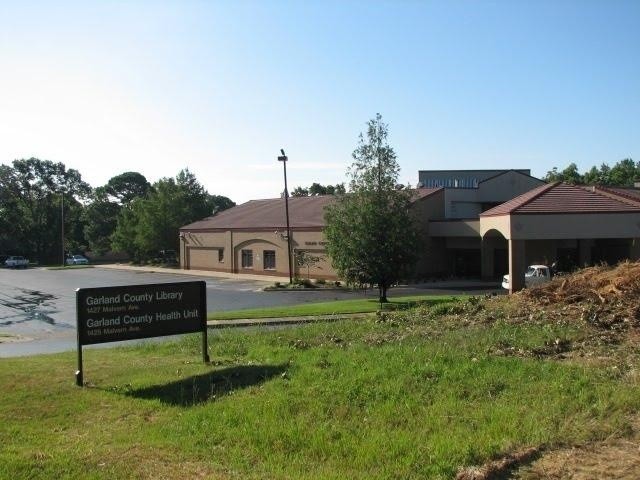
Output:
[65,255,89,266]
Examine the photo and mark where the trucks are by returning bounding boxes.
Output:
[502,264,571,290]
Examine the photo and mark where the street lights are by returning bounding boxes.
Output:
[277,148,293,284]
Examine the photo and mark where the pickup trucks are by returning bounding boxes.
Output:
[4,256,29,269]
